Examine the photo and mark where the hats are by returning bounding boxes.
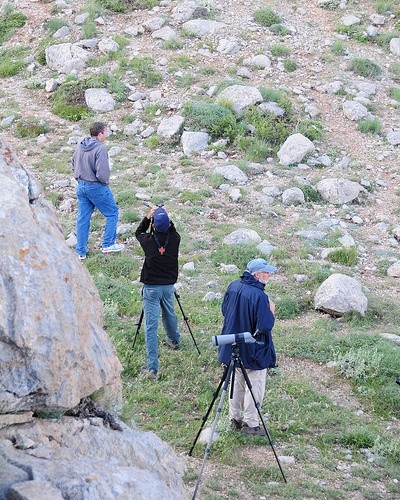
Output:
[154,208,169,232]
[247,258,277,273]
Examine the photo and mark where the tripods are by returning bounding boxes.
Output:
[131,292,201,356]
[189,342,288,500]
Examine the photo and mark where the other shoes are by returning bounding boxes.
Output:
[79,249,90,260]
[140,366,156,378]
[162,338,179,349]
[230,418,266,436]
[102,244,123,253]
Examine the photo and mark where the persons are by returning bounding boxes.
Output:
[217,258,276,436]
[135,206,181,379]
[71,122,125,260]
